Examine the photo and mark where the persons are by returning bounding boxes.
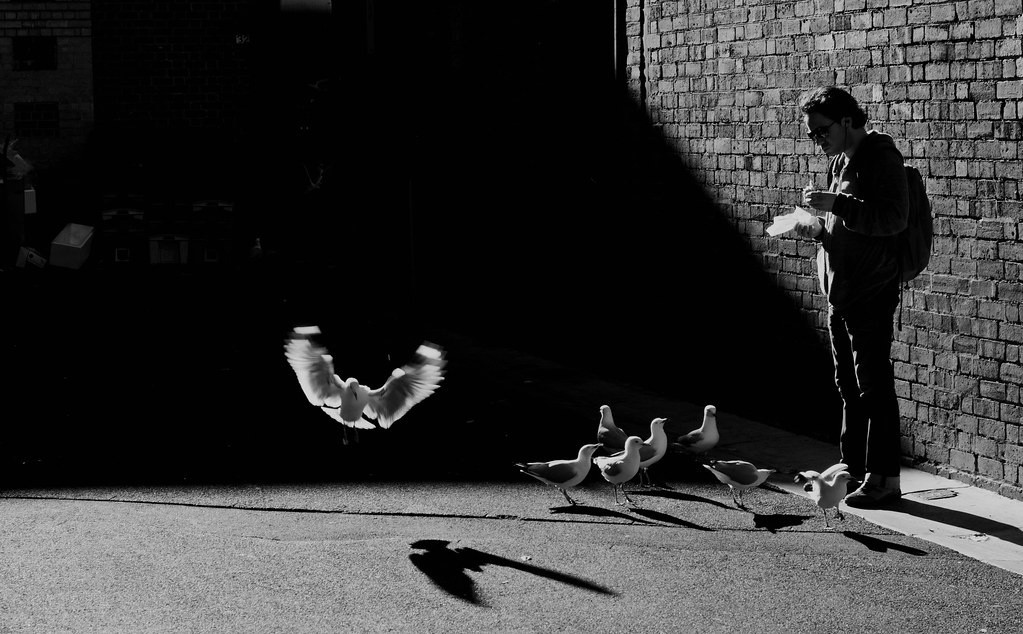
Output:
[793,85,912,508]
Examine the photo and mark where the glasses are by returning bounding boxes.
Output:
[807,117,841,141]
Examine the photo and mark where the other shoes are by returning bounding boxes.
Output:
[845,473,901,509]
[804,476,864,493]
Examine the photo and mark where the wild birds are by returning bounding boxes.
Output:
[515,443,603,508]
[596,405,628,453]
[284,326,448,446]
[593,435,652,505]
[703,459,777,509]
[795,463,854,528]
[610,417,669,489]
[677,404,720,453]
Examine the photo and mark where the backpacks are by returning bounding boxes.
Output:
[828,141,933,282]
